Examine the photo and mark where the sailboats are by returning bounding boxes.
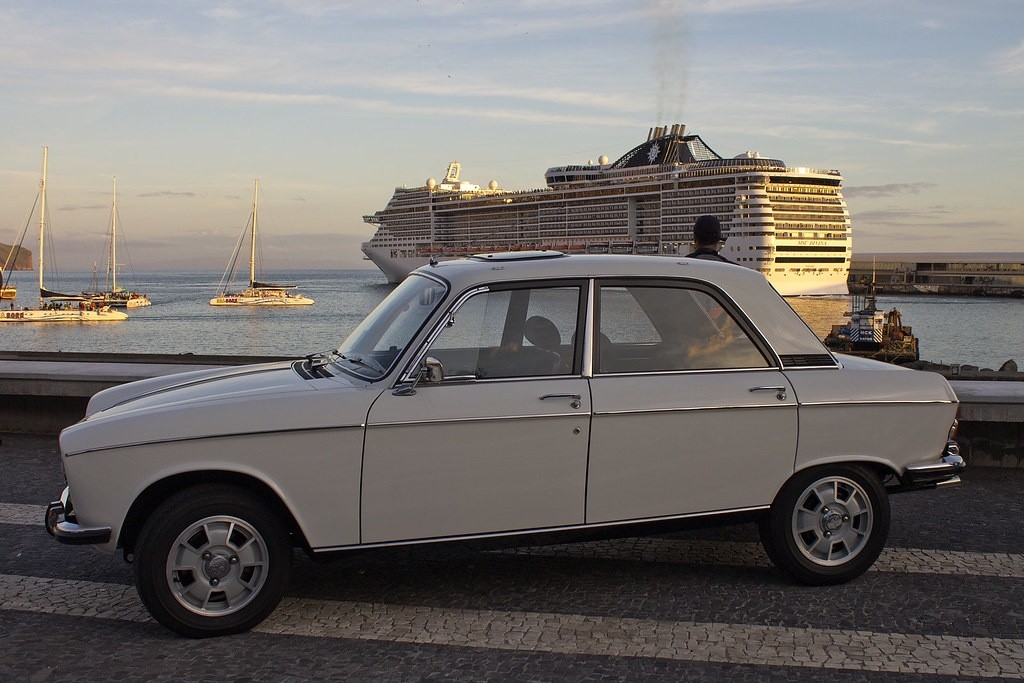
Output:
[47,175,153,310]
[208,178,316,307]
[0,146,130,324]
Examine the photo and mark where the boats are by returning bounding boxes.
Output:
[612,246,631,254]
[569,245,586,254]
[483,245,494,253]
[817,254,920,367]
[494,245,509,252]
[636,243,659,254]
[443,246,454,256]
[589,246,608,254]
[468,246,482,254]
[551,245,568,254]
[511,243,521,251]
[417,249,431,258]
[535,244,551,251]
[431,247,443,257]
[455,245,469,257]
[522,244,535,251]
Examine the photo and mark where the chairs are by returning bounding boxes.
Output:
[504,316,611,377]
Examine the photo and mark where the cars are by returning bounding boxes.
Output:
[44,254,968,642]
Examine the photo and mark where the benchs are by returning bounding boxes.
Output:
[650,333,766,369]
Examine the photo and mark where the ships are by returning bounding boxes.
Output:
[359,123,855,299]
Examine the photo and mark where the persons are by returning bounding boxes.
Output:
[50,300,53,307]
[685,215,740,265]
[10,302,14,310]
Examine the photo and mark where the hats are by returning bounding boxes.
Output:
[694,215,721,237]
